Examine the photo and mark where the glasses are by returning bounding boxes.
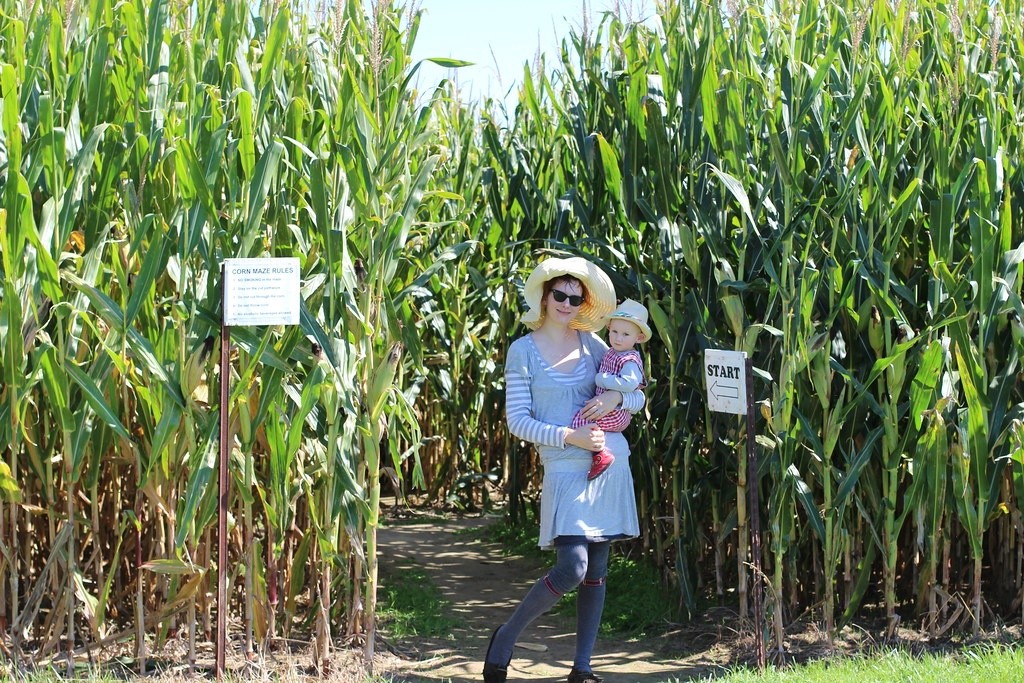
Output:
[550,289,585,306]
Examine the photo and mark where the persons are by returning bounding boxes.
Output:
[483,256,647,682]
[571,298,651,482]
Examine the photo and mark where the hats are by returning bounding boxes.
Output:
[605,298,652,343]
[520,257,618,332]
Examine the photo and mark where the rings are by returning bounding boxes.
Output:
[598,409,601,414]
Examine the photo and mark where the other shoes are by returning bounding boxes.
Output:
[483,623,513,683]
[567,666,601,683]
[587,449,614,481]
